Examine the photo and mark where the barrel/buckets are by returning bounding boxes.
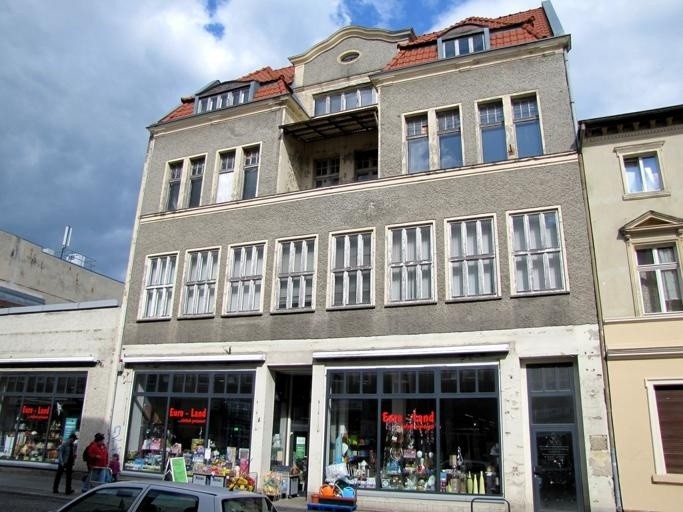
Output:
[342,487,355,498]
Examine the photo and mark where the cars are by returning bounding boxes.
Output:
[54,480,277,512]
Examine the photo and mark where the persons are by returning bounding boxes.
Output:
[51,432,79,495]
[81,432,123,491]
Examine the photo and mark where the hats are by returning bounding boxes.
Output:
[95,433,105,439]
[69,434,79,439]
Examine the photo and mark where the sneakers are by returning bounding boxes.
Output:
[67,490,74,496]
[53,490,60,494]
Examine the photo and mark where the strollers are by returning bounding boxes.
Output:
[81,465,114,497]
